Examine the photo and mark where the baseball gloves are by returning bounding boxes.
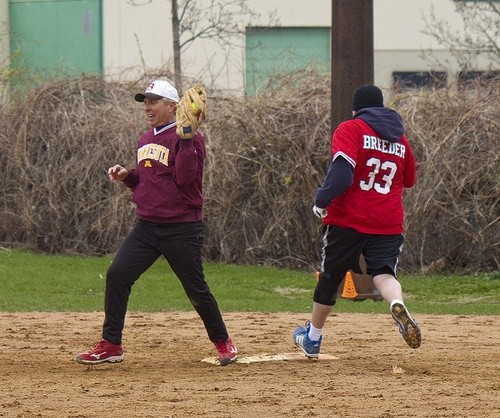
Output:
[173,82,208,140]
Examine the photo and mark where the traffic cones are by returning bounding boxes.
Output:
[341,272,358,298]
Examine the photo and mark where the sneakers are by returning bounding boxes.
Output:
[212,337,238,365]
[76,338,124,364]
[389,298,422,349]
[293,320,323,359]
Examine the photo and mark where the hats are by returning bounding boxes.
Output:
[134,79,180,104]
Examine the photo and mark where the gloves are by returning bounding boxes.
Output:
[312,205,328,218]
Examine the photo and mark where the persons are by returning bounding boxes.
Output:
[293,84,421,357]
[76,80,237,364]
